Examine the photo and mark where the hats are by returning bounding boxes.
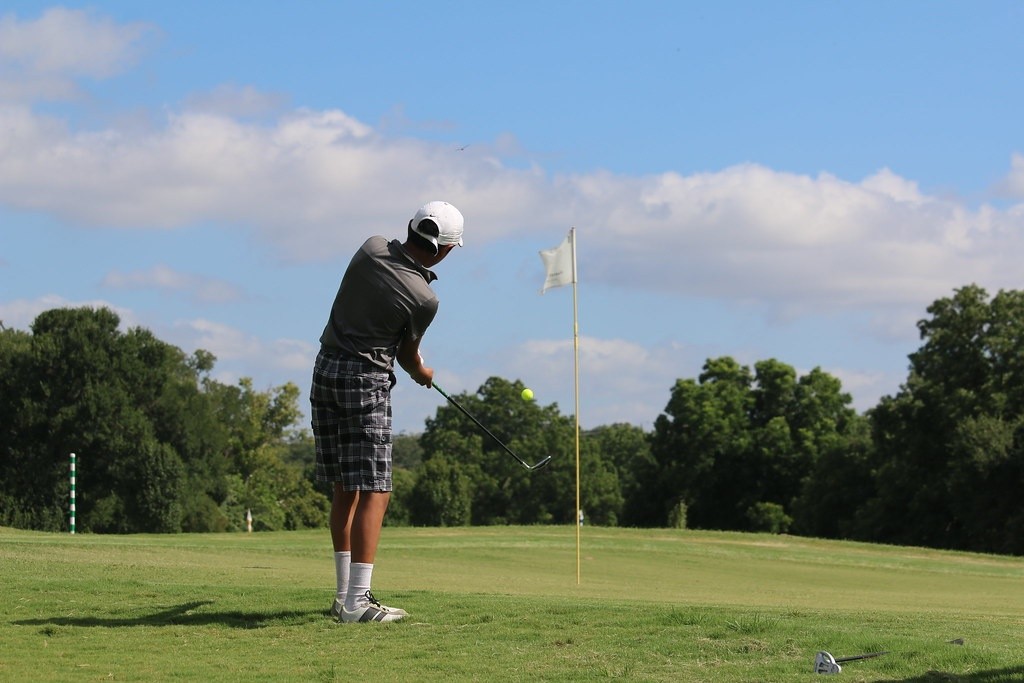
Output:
[412,201,464,256]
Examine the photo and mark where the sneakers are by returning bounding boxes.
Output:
[340,589,411,624]
[330,593,347,619]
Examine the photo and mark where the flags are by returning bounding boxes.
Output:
[540,231,574,291]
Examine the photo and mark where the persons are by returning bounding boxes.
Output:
[308,201,464,625]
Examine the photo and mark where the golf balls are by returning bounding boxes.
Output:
[430,380,553,473]
[521,388,534,400]
